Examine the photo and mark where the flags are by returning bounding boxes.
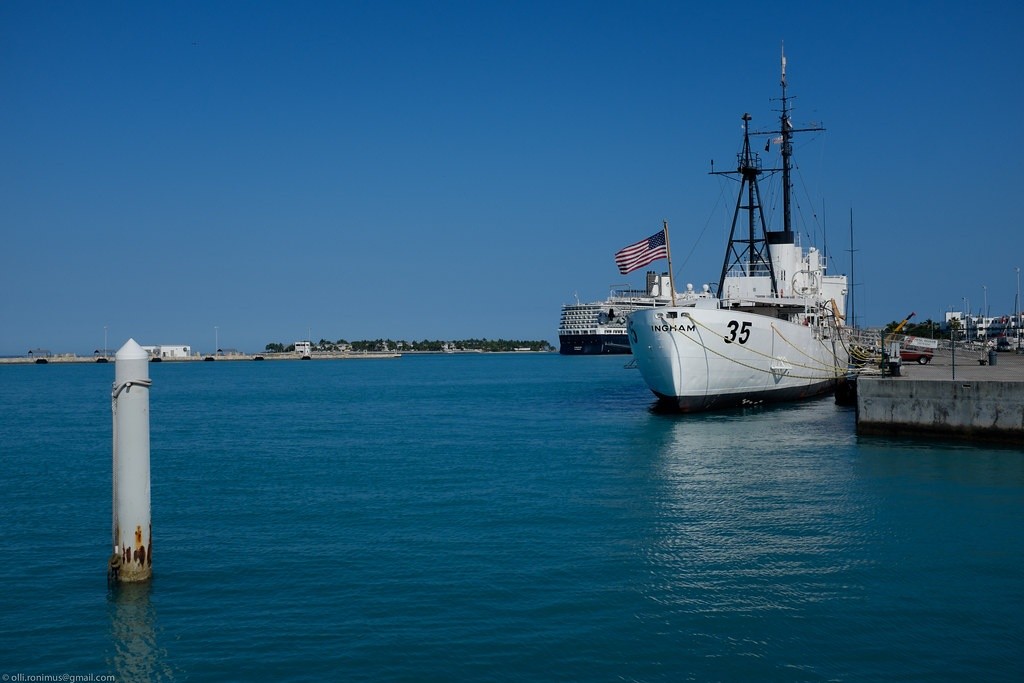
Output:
[615,229,668,275]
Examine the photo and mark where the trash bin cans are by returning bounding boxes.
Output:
[888,356,902,377]
[987,351,998,366]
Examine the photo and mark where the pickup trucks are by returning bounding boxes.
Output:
[877,312,933,365]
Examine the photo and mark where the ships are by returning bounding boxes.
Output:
[557,270,718,355]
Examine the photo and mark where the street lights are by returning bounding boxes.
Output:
[308,328,310,342]
[215,326,219,358]
[981,285,988,341]
[962,297,970,341]
[104,326,106,357]
[1014,267,1020,328]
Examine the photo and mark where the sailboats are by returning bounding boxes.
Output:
[623,37,855,414]
